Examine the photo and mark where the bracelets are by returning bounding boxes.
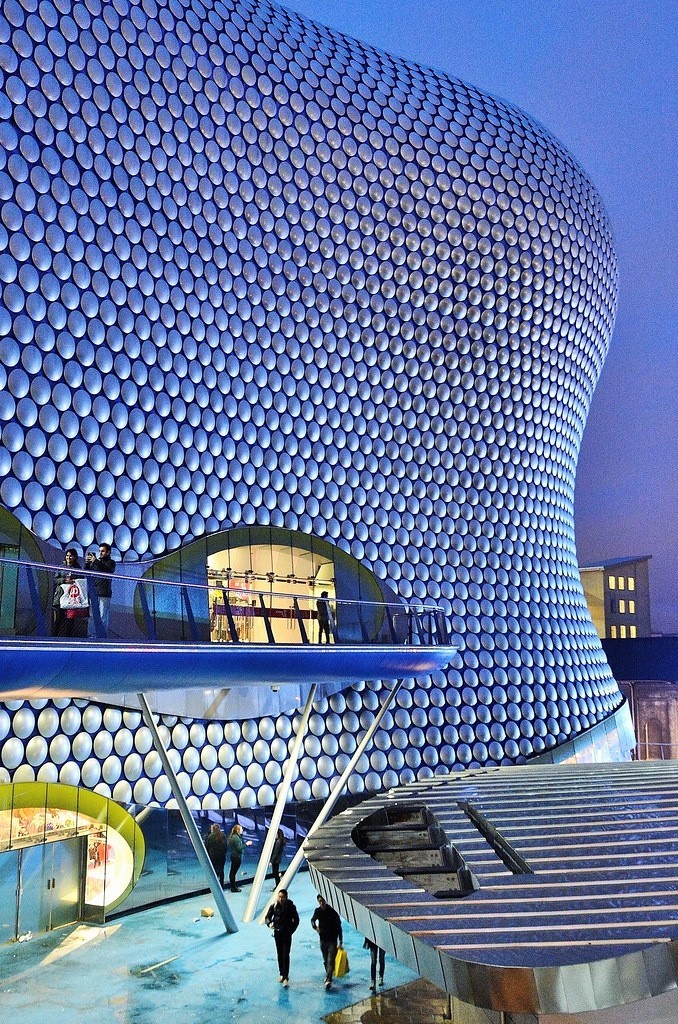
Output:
[246,845,247,847]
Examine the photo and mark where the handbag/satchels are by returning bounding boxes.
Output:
[340,948,350,973]
[333,945,346,978]
[363,937,370,949]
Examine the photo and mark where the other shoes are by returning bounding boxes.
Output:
[278,975,283,981]
[282,979,288,986]
[378,977,383,986]
[324,977,331,990]
[369,979,376,990]
[270,888,275,892]
[231,888,241,892]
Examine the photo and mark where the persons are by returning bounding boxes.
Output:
[363,937,387,990]
[269,828,285,892]
[315,591,332,645]
[52,548,84,637]
[264,890,299,988]
[205,823,227,891]
[84,543,116,638]
[310,895,343,989]
[227,824,254,892]
